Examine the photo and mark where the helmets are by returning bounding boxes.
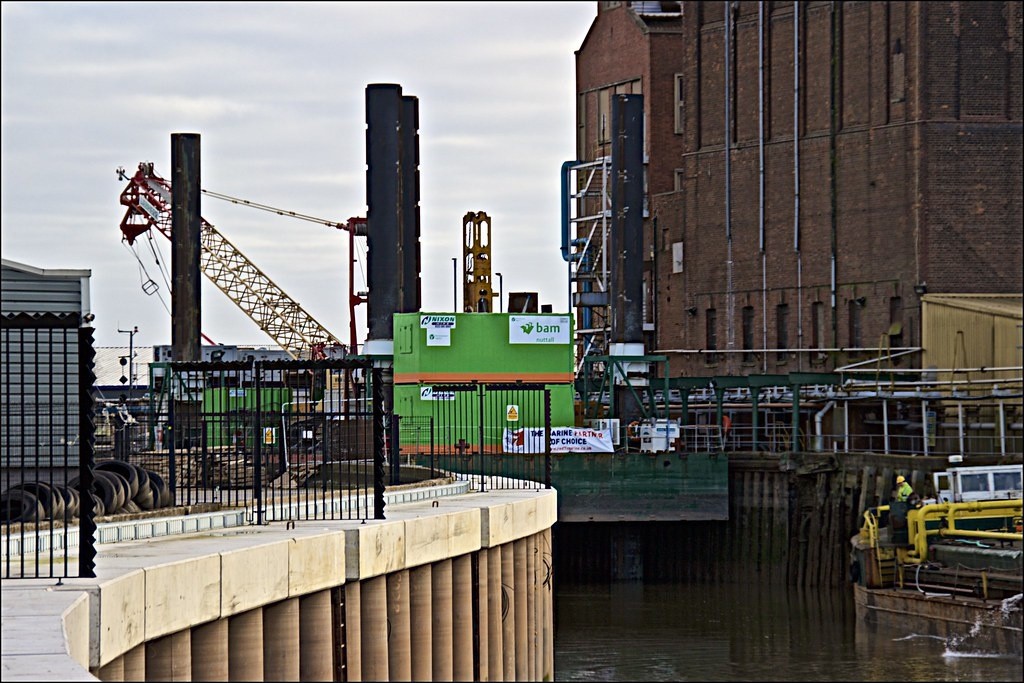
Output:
[896,476,905,483]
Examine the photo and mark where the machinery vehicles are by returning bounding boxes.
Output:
[116,161,370,452]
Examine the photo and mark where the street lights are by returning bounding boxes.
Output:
[452,258,457,312]
[495,273,502,313]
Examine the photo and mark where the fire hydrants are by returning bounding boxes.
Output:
[454,439,470,454]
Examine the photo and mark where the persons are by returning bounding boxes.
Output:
[894,476,913,503]
[349,371,362,413]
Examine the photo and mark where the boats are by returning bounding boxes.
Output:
[854,455,1023,654]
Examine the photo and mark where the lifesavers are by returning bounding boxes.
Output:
[848,561,860,583]
[1,459,170,524]
[627,421,642,440]
